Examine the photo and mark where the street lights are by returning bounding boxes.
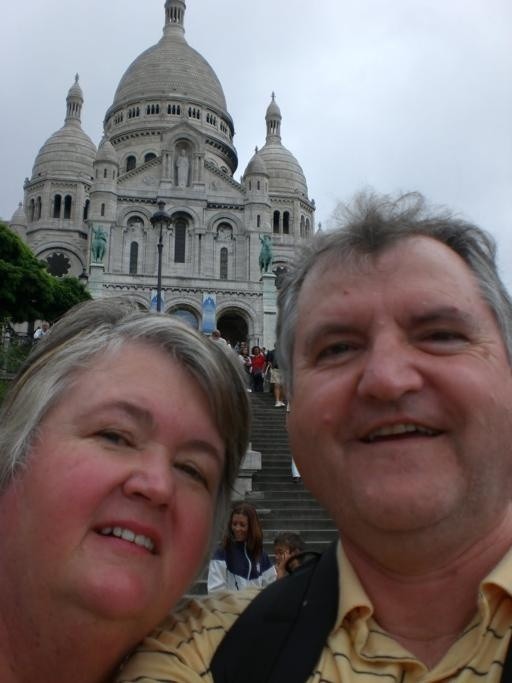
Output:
[148,198,175,314]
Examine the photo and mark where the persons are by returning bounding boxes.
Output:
[229,337,288,408]
[33,321,51,340]
[258,233,271,266]
[0,292,254,682]
[206,502,279,599]
[261,531,308,588]
[207,329,227,344]
[91,224,108,260]
[175,147,191,188]
[101,177,511,682]
